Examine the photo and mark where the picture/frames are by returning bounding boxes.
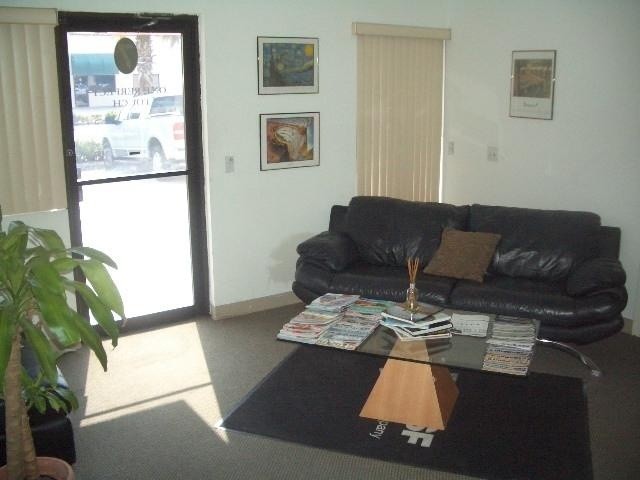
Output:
[507,46,559,123]
[259,110,321,173]
[255,34,321,97]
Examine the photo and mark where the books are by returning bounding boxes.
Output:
[276,293,538,375]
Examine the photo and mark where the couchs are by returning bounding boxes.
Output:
[292,193,629,344]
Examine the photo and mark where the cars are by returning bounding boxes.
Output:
[101,92,186,171]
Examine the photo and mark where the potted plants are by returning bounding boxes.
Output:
[0,216,130,479]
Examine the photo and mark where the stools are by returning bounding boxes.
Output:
[1,342,85,470]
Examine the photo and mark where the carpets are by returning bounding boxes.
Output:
[219,343,595,479]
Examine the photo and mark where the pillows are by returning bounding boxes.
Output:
[422,222,501,284]
